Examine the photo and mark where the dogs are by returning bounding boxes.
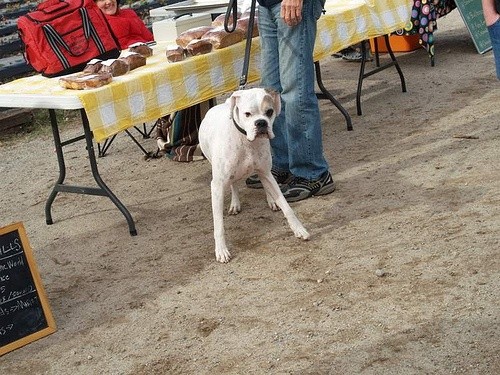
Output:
[196,87,310,263]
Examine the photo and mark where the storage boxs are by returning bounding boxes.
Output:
[152,12,212,44]
[370,34,424,53]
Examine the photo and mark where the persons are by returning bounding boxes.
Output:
[95,0,154,48]
[482,0,500,79]
[245,0,335,202]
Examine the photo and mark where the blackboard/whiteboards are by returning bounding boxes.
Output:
[0,220,58,359]
[455,0,494,55]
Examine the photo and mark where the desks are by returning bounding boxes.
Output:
[0,0,457,237]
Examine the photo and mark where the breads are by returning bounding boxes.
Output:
[166,7,259,61]
[59,43,153,90]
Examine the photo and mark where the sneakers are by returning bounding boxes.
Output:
[246,168,292,188]
[278,170,335,201]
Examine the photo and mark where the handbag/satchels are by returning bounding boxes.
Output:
[17,0,120,78]
